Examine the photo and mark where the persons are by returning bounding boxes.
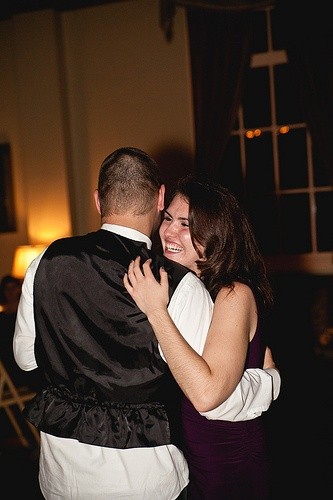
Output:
[0,274,38,451]
[12,148,282,500]
[123,180,272,500]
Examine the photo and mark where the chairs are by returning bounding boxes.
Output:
[0,361,39,447]
